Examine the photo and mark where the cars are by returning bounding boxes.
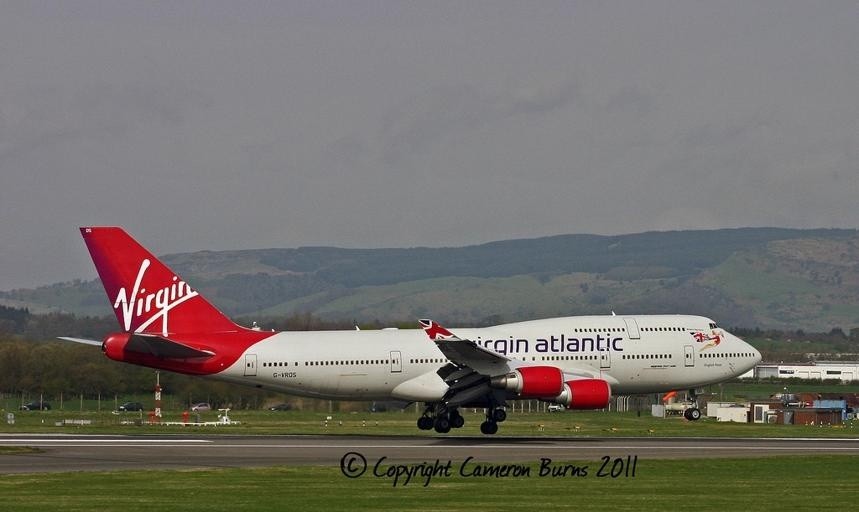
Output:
[268,404,289,412]
[19,399,51,411]
[190,403,211,412]
[119,401,144,412]
[368,405,386,413]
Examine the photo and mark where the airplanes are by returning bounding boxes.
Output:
[56,226,763,435]
